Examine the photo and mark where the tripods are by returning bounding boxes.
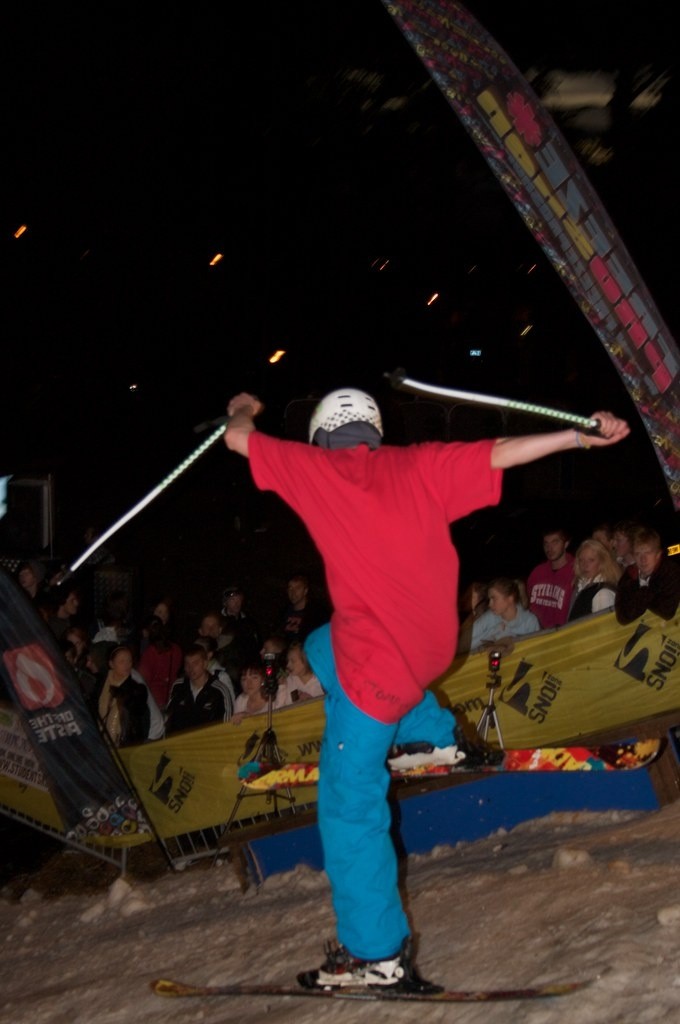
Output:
[473,670,506,751]
[208,695,298,877]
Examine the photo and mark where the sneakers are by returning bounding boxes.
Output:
[388,738,460,772]
[296,936,403,987]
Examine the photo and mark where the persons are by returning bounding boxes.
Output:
[568,538,620,623]
[471,581,541,648]
[0,518,680,753]
[274,575,322,640]
[223,389,632,992]
[170,645,233,730]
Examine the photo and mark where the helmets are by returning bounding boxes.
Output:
[311,388,382,444]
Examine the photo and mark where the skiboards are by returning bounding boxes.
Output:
[150,734,670,1002]
